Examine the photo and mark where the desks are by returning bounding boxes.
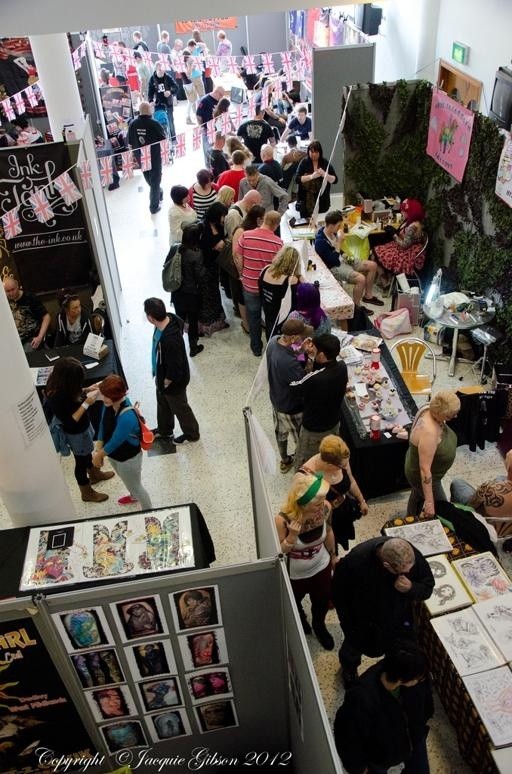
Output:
[423,294,496,375]
[318,217,405,247]
[381,502,512,773]
[24,340,128,440]
[340,327,419,501]
[0,504,216,601]
[281,240,355,329]
[202,47,312,191]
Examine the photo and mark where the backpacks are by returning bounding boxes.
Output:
[50,415,70,456]
[119,401,154,450]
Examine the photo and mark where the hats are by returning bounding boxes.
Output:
[287,135,296,144]
[312,334,340,352]
[281,319,314,335]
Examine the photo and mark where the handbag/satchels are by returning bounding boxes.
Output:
[420,266,456,303]
[279,285,292,325]
[344,494,362,521]
[162,253,182,292]
[217,238,239,280]
[374,308,412,338]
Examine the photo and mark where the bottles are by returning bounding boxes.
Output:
[371,349,381,369]
[370,416,381,441]
[344,216,348,233]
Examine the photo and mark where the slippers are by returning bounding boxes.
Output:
[241,321,250,334]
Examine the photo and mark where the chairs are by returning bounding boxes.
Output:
[390,337,436,401]
[481,517,512,550]
[312,240,348,290]
[387,234,429,298]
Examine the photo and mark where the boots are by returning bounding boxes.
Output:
[78,479,108,502]
[87,466,114,485]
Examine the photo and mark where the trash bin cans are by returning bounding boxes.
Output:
[396,279,423,326]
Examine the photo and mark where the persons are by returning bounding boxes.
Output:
[334,649,435,774]
[296,436,370,556]
[451,449,512,538]
[331,536,435,693]
[126,604,156,635]
[184,591,211,626]
[98,687,128,717]
[142,643,164,674]
[403,392,463,516]
[274,471,340,651]
[0,27,429,513]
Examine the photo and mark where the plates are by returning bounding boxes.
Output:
[350,332,384,353]
[382,405,399,420]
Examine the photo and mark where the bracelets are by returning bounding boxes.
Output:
[283,538,295,548]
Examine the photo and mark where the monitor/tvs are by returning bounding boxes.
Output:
[354,4,383,35]
[487,65,512,131]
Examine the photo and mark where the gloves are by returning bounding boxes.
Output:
[384,225,397,237]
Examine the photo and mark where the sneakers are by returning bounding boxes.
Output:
[300,611,311,636]
[119,496,139,504]
[160,189,162,201]
[313,619,333,649]
[362,296,384,306]
[152,207,161,214]
[342,666,358,689]
[254,352,262,356]
[361,305,374,315]
[280,454,295,474]
[152,428,172,437]
[173,434,192,445]
[190,345,204,357]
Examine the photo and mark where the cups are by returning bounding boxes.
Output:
[396,213,401,223]
